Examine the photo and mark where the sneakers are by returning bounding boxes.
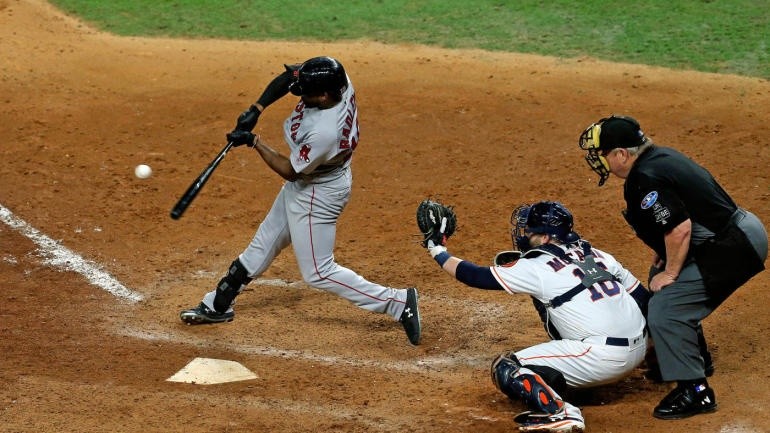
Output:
[653,382,717,419]
[399,287,421,346]
[519,416,585,432]
[181,302,235,326]
[705,355,716,376]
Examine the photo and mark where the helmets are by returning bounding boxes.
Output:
[289,56,349,96]
[527,202,579,242]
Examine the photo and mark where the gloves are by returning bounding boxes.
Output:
[233,103,262,133]
[226,131,257,147]
[427,239,451,268]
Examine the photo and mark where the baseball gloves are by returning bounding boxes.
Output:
[409,193,463,250]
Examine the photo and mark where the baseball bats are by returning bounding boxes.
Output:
[170,141,236,220]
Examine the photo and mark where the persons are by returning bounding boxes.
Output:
[579,115,770,420]
[178,55,420,347]
[415,199,653,433]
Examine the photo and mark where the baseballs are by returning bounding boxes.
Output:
[134,164,152,179]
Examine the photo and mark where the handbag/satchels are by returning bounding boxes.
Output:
[694,225,766,305]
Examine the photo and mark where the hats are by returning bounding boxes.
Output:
[598,115,645,147]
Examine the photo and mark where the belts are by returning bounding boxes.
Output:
[581,327,647,346]
[702,208,747,247]
